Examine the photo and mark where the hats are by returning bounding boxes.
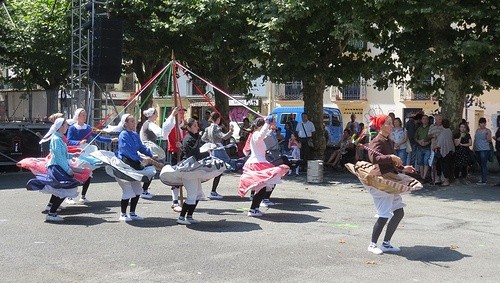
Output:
[369,115,388,131]
[177,106,187,111]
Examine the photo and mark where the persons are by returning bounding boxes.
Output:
[162,118,226,224]
[274,127,285,151]
[67,108,118,203]
[169,108,188,210]
[201,111,234,198]
[17,114,86,209]
[325,112,500,187]
[288,134,300,176]
[296,113,316,172]
[238,114,290,217]
[238,118,252,139]
[285,113,299,136]
[26,118,83,220]
[140,106,178,198]
[90,114,165,221]
[346,114,422,255]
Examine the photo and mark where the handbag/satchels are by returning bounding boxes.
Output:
[404,128,412,153]
[308,138,314,147]
[488,141,493,162]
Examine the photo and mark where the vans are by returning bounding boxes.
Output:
[264,104,344,147]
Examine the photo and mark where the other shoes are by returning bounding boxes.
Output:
[381,244,400,252]
[368,244,383,254]
[209,194,223,199]
[45,206,61,211]
[176,219,191,224]
[186,219,199,224]
[248,195,274,217]
[419,176,487,186]
[66,198,76,205]
[172,205,182,211]
[59,205,65,209]
[79,198,91,202]
[129,214,144,220]
[46,215,64,221]
[140,192,152,199]
[295,172,299,176]
[119,215,132,221]
[287,173,292,176]
